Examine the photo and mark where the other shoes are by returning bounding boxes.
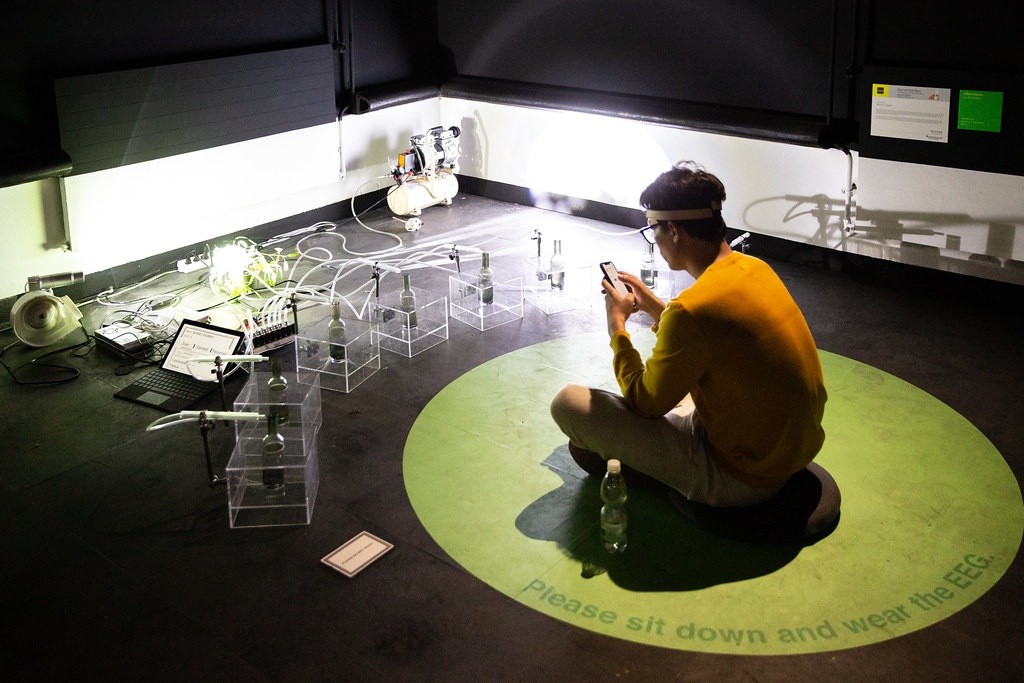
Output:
[569,440,642,475]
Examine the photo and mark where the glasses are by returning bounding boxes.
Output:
[638,221,668,244]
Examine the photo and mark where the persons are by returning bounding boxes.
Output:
[550,168,828,515]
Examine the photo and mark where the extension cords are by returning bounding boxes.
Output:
[176,251,216,274]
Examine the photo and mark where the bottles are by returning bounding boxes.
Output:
[262,412,285,488]
[478,252,493,305]
[599,459,627,556]
[328,301,347,361]
[400,272,417,329]
[641,241,654,290]
[267,359,290,426]
[551,240,564,290]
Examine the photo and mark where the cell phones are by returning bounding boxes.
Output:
[600,261,636,307]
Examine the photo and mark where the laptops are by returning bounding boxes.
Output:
[113,320,245,416]
